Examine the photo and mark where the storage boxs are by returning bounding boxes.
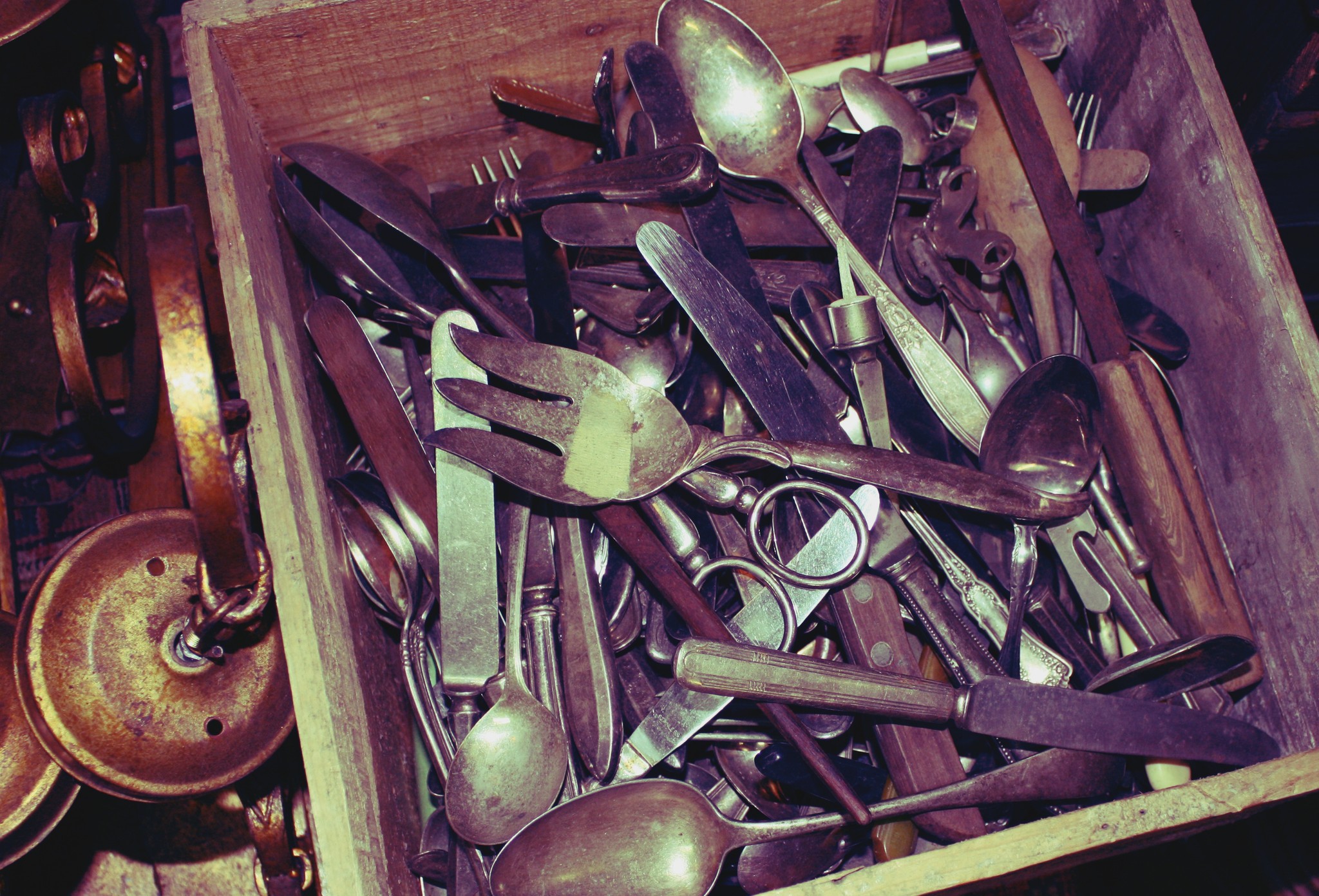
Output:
[176,0,1319,895]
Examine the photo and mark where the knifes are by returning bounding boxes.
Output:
[632,222,1014,687]
[432,308,504,896]
[578,485,882,788]
[670,636,1284,768]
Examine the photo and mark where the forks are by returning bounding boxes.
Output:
[1066,92,1103,150]
[424,321,1070,522]
[471,145,522,185]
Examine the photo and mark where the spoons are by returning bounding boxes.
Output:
[283,0,1290,894]
